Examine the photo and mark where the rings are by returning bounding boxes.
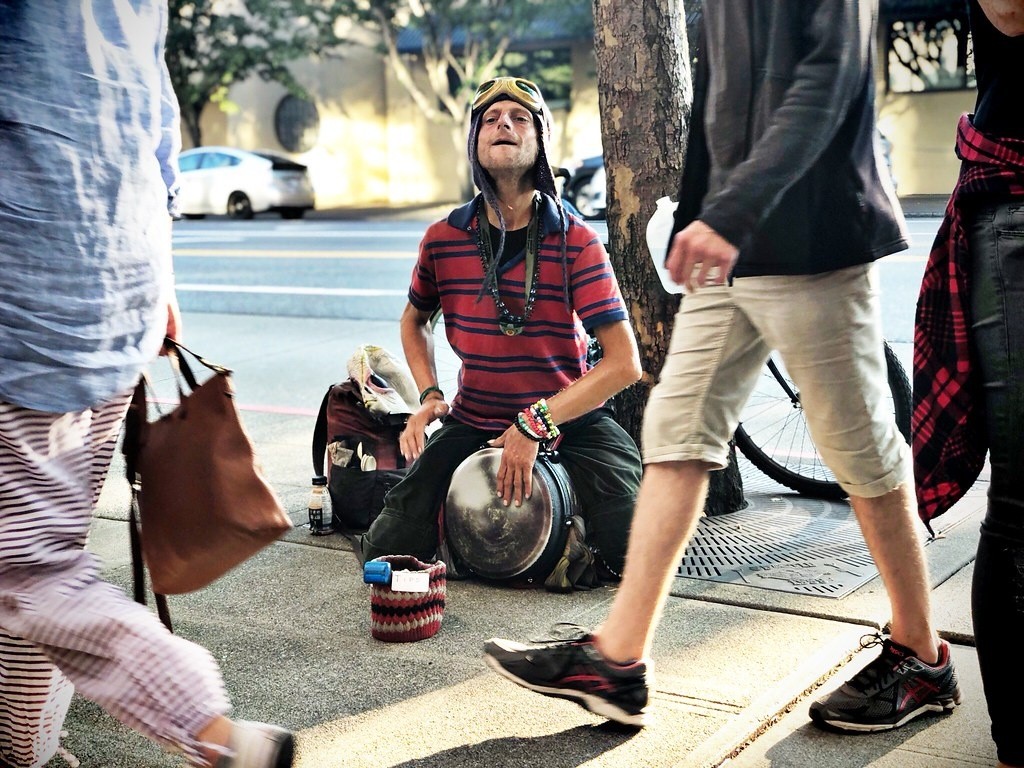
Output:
[671,245,689,256]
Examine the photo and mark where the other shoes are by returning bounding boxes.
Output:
[216,719,294,768]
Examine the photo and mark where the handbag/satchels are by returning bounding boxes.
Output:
[120,338,294,634]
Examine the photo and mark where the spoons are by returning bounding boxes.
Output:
[357,441,376,471]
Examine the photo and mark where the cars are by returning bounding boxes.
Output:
[568,155,609,222]
[174,147,316,219]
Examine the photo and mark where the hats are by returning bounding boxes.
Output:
[467,85,570,304]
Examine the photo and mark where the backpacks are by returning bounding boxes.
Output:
[312,376,444,545]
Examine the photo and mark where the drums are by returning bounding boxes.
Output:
[446,443,579,587]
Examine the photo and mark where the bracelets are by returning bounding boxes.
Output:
[418,385,444,403]
[514,397,561,441]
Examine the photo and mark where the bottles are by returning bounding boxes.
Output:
[309,476,334,533]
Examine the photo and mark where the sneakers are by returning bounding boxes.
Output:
[808,634,962,732]
[485,623,651,726]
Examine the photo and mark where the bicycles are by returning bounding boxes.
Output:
[733,329,913,504]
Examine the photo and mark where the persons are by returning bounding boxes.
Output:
[0,1,297,768]
[363,78,644,581]
[485,0,961,735]
[914,2,1024,767]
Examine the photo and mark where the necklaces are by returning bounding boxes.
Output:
[477,190,543,338]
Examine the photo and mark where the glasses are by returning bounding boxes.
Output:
[472,76,545,115]
